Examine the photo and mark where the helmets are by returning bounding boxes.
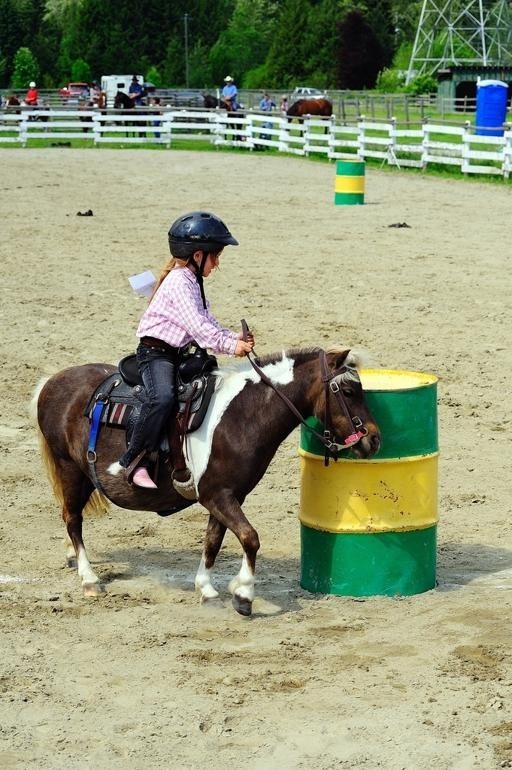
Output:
[168,211,239,259]
[92,80,97,86]
[29,82,36,88]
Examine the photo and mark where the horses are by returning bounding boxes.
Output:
[78,92,108,138]
[29,338,383,617]
[113,90,153,138]
[285,98,332,137]
[2,96,54,134]
[200,93,244,150]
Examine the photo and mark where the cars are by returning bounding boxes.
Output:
[292,86,325,100]
[58,83,89,104]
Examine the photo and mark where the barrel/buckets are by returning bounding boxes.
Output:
[299,368,438,595]
[334,160,366,205]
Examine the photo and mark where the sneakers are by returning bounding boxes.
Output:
[132,467,158,490]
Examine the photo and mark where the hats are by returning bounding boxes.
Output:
[223,76,234,82]
[132,77,139,81]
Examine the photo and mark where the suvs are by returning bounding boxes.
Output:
[147,87,213,110]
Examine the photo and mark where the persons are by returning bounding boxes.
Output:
[106,213,255,492]
[23,82,38,121]
[89,80,103,108]
[280,98,288,112]
[149,97,162,145]
[258,94,277,129]
[221,76,238,111]
[128,76,143,105]
[59,86,70,105]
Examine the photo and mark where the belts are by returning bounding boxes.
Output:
[140,337,190,354]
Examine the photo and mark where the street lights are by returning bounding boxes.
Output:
[183,13,190,89]
